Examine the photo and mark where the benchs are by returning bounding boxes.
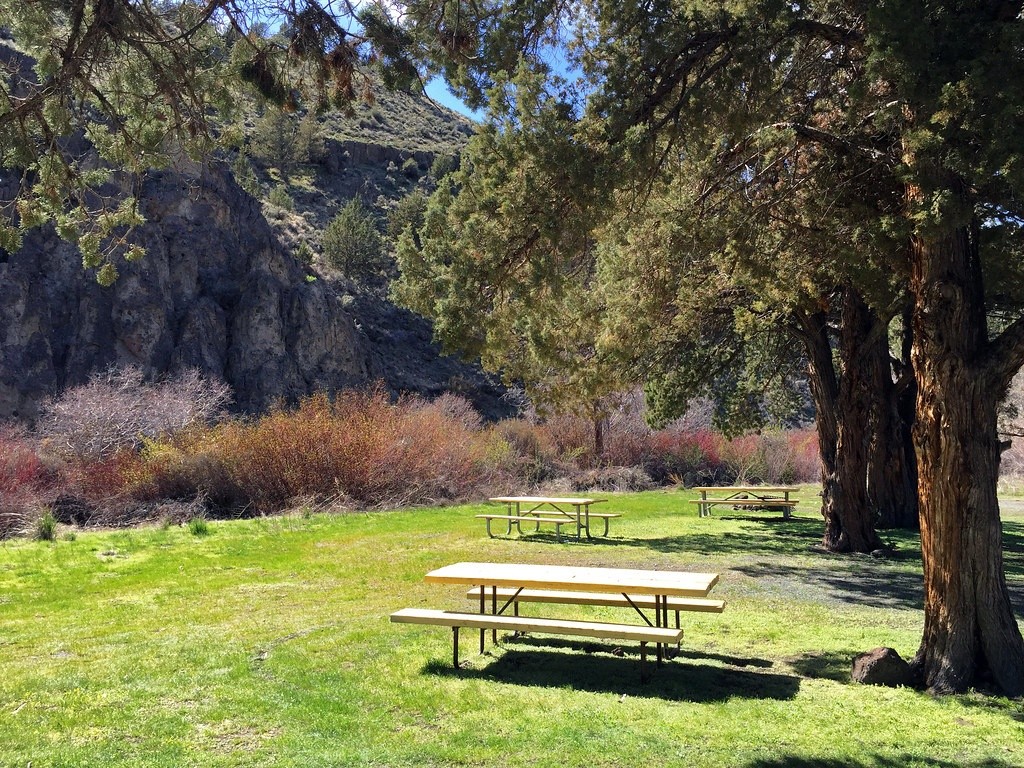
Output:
[475,515,578,542]
[520,511,621,539]
[467,588,726,655]
[390,608,683,685]
[689,498,799,517]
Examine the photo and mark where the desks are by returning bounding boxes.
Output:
[693,487,800,514]
[425,561,720,652]
[489,497,608,534]
